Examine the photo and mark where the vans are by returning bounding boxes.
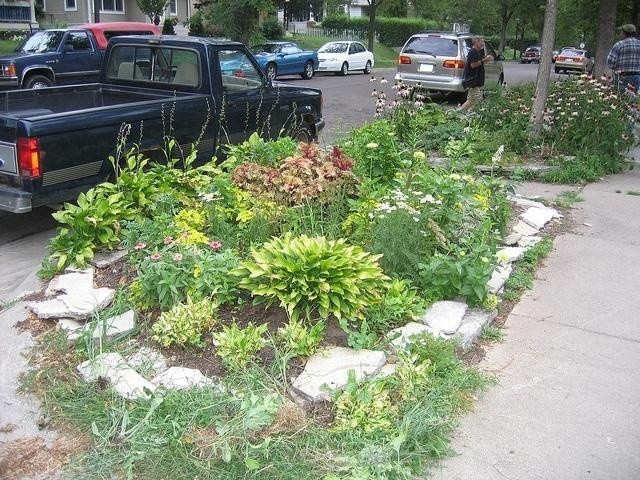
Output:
[394,30,505,101]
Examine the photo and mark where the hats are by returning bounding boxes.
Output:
[617,24,637,34]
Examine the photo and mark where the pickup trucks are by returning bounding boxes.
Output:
[1,31,324,214]
[0,20,161,87]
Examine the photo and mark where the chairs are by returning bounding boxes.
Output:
[172,63,199,86]
[117,62,144,80]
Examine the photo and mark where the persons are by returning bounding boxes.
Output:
[606,23,640,125]
[456,35,495,115]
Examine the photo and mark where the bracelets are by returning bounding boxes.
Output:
[481,59,484,64]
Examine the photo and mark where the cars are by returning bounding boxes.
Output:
[555,48,595,74]
[317,41,375,75]
[519,46,559,63]
[218,42,319,79]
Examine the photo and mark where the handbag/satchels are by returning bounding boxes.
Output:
[462,77,476,90]
